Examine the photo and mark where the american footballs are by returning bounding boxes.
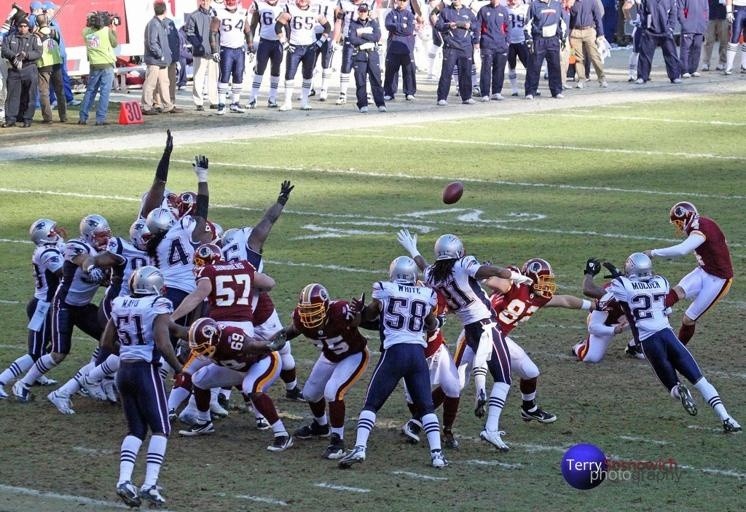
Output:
[443,182,463,204]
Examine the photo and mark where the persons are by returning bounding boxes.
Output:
[643,201,734,345]
[337,256,449,470]
[109,265,191,506]
[0,1,746,130]
[0,129,608,454]
[600,252,744,435]
[261,281,371,460]
[572,258,659,363]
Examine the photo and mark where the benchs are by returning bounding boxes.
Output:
[53,41,145,94]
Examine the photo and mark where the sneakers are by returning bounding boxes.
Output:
[624,344,646,359]
[678,384,698,416]
[722,418,742,432]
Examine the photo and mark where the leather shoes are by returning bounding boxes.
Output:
[2,99,218,127]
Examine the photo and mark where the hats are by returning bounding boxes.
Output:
[357,4,370,11]
[16,19,29,29]
[42,1,59,10]
[30,1,43,10]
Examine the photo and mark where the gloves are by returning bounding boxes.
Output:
[584,256,601,277]
[211,34,339,62]
[603,262,623,279]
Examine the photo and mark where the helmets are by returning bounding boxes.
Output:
[390,235,557,300]
[624,252,652,281]
[669,201,700,233]
